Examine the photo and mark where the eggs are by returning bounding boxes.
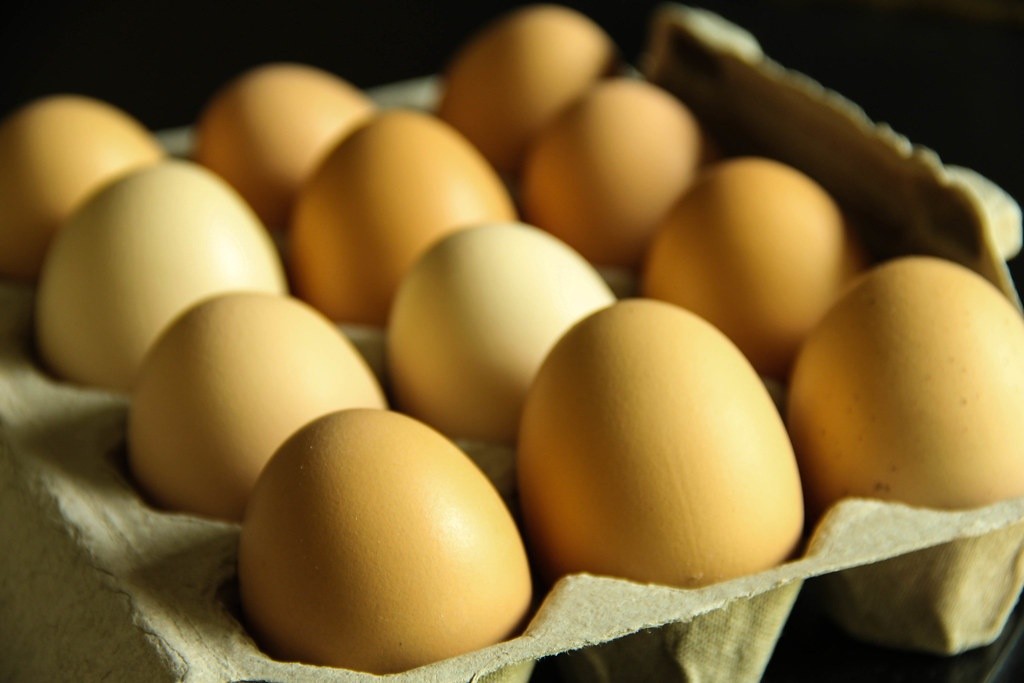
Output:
[0,7,1024,675]
